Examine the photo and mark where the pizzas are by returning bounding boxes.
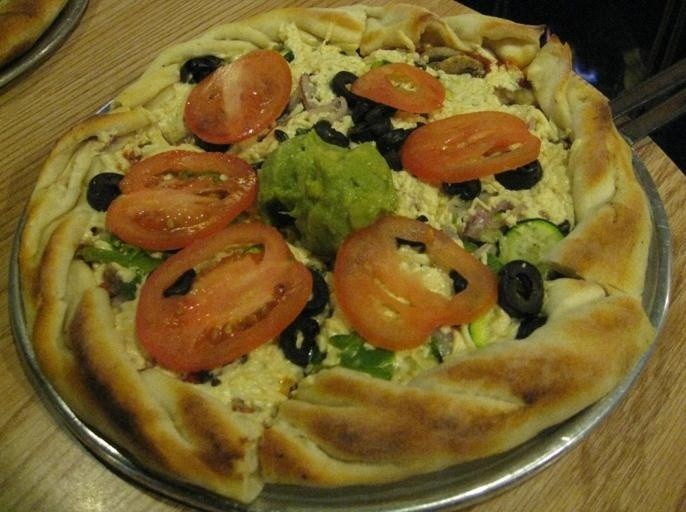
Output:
[0,1,69,68]
[16,1,656,505]
